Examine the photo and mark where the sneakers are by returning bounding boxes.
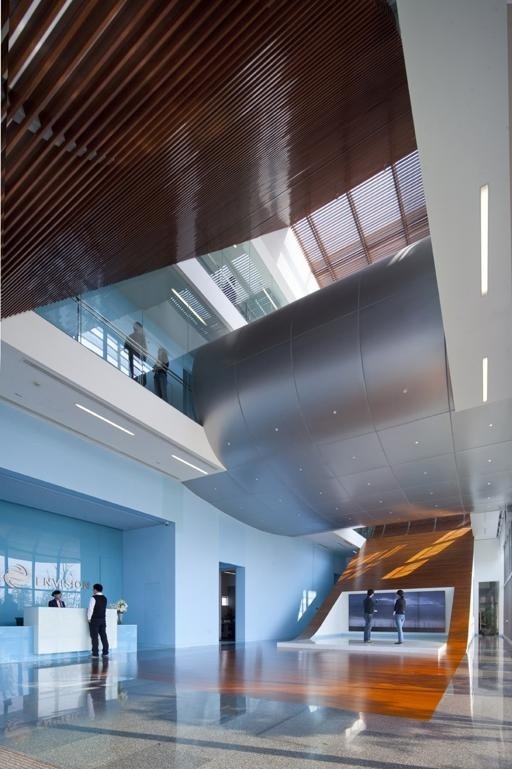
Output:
[89,654,99,658]
[103,653,108,656]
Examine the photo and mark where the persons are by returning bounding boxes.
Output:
[49,590,66,608]
[362,588,378,643]
[152,347,169,402]
[392,589,407,644]
[124,321,148,386]
[87,583,110,659]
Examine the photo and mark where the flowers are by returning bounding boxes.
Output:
[110,599,129,614]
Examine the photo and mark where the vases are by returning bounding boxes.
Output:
[117,612,123,625]
